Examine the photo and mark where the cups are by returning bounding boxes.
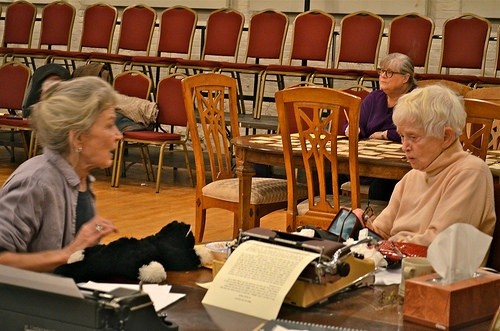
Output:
[399,256,436,297]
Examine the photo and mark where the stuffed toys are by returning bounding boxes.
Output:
[54,220,213,283]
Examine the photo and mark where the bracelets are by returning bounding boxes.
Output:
[382,131,385,140]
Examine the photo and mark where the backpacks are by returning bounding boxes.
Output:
[115,115,153,134]
[21,65,113,119]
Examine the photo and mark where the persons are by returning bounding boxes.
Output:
[343,52,418,202]
[365,83,496,266]
[0,76,124,271]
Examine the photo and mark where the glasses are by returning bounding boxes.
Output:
[362,199,374,222]
[377,68,404,78]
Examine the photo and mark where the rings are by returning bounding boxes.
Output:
[95,224,102,231]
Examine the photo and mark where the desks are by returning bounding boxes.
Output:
[229,131,500,242]
[162,268,500,331]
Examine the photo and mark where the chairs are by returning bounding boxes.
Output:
[0,0,500,244]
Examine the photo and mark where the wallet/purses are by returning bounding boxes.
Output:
[368,240,428,263]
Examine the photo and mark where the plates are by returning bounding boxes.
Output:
[205,240,231,260]
[368,241,429,268]
